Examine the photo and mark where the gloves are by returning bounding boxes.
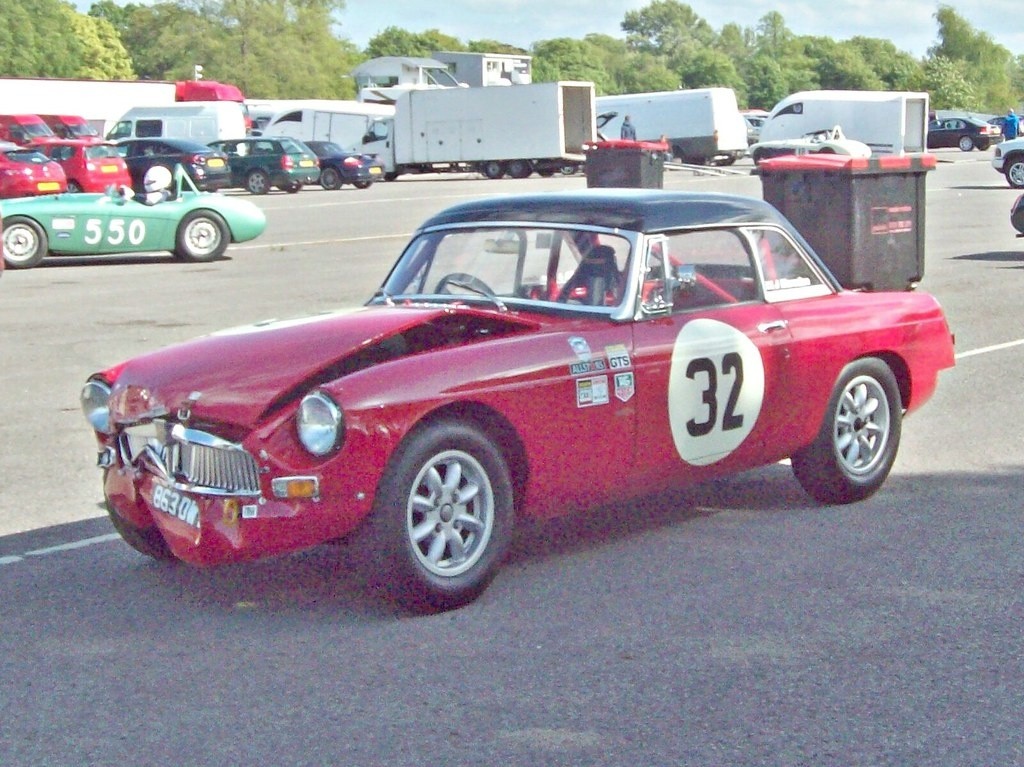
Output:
[132,194,146,204]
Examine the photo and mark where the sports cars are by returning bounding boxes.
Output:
[0,161,267,270]
[80,188,958,614]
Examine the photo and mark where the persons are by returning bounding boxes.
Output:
[1000,108,1020,142]
[620,116,636,141]
[651,136,674,171]
[119,165,173,206]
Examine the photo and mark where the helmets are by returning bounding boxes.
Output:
[144,165,172,193]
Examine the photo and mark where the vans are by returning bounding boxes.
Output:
[104,99,248,145]
[0,114,133,200]
[247,102,394,163]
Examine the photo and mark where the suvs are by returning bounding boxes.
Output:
[204,136,322,196]
[114,136,233,193]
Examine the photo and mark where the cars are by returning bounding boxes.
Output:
[285,139,385,191]
[1010,192,1024,237]
[987,114,1024,136]
[741,111,767,145]
[991,136,1024,189]
[927,116,1004,152]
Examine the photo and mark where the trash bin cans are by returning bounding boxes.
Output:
[584,140,670,189]
[750,152,938,291]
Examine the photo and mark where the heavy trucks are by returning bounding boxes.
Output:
[350,79,599,182]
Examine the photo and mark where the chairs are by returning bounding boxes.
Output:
[228,145,239,156]
[557,247,624,308]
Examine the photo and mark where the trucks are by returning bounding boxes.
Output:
[751,90,930,168]
